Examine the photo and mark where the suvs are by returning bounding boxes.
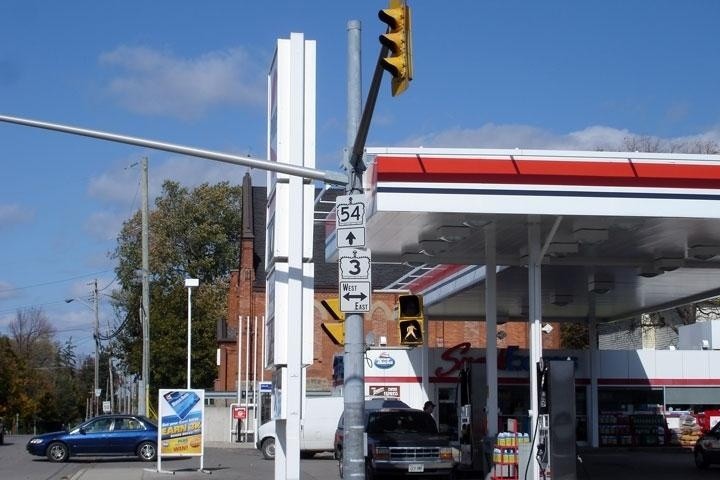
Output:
[337,409,455,480]
[334,399,410,458]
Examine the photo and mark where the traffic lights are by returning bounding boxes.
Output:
[397,292,425,344]
[379,0,414,97]
[320,296,345,348]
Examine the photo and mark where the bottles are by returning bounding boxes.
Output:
[493,448,520,464]
[599,404,664,446]
[497,432,529,447]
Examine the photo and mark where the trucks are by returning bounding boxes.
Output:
[255,393,394,461]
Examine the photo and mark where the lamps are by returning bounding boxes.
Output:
[520,247,720,315]
[402,226,471,268]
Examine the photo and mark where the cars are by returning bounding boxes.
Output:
[27,413,157,464]
[695,419,720,467]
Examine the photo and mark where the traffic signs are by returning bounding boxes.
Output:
[339,281,371,314]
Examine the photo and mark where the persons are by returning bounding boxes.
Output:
[423,401,437,417]
[0,404,7,436]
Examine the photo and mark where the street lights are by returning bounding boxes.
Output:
[66,278,101,418]
[184,278,199,391]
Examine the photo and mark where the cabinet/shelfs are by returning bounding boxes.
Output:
[491,417,518,480]
